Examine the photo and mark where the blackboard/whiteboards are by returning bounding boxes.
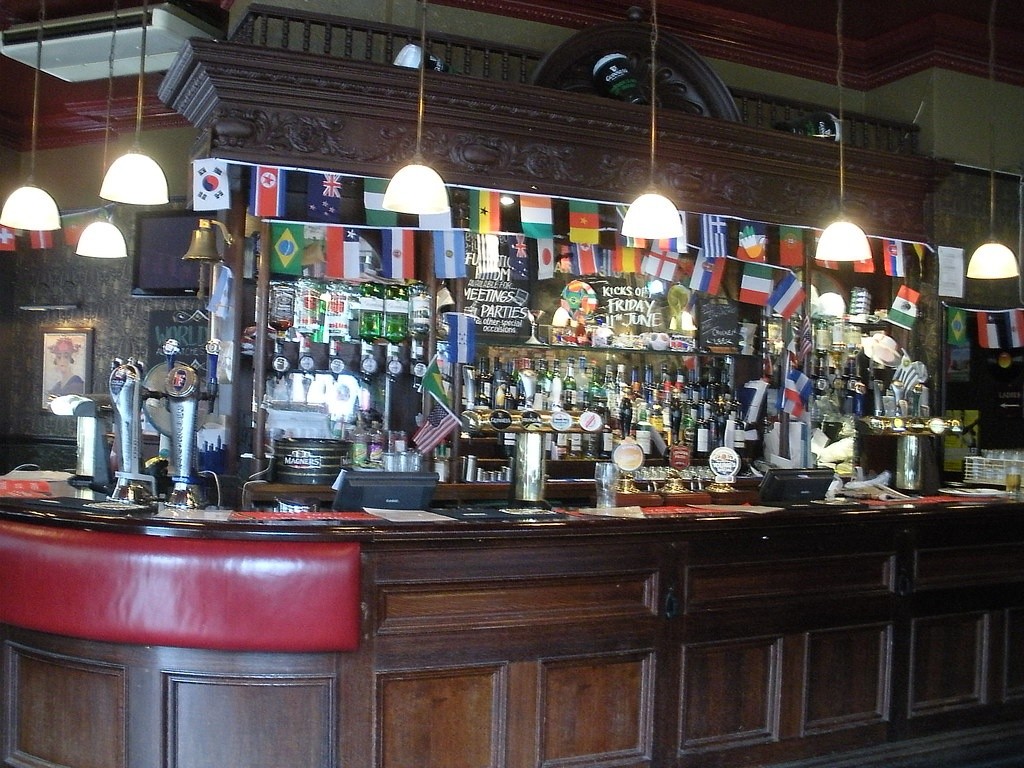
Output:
[697,293,741,353]
[463,232,538,343]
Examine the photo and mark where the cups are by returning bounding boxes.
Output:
[973,448,1024,499]
[626,466,717,479]
[594,463,618,510]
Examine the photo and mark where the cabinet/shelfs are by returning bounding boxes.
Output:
[806,230,942,479]
[258,219,431,491]
[451,229,763,483]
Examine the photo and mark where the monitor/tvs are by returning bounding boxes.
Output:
[330,471,440,512]
[758,468,835,502]
[130,210,217,298]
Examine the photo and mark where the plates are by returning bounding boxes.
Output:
[938,488,1013,497]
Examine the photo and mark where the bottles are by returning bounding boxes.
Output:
[270,279,430,365]
[460,357,746,458]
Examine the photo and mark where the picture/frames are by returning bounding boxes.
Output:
[35,325,95,412]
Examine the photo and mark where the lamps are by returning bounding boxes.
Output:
[1,0,62,232]
[100,1,170,206]
[383,0,451,216]
[622,1,685,241]
[967,1,1020,280]
[77,1,128,259]
[814,0,872,262]
